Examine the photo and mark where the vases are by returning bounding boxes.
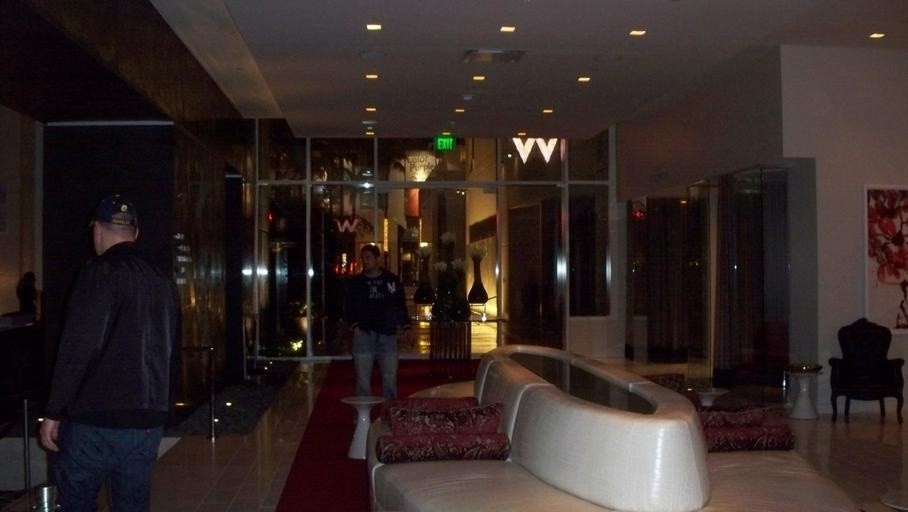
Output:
[413,245,489,320]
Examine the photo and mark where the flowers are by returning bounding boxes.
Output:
[440,231,455,244]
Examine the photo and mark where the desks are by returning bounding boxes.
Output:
[342,396,386,459]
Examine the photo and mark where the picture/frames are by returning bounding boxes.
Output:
[863,182,908,336]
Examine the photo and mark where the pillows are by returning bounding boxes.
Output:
[685,389,792,449]
[369,395,510,461]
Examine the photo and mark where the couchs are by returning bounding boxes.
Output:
[366,344,857,512]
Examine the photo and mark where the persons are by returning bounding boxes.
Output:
[339,243,416,401]
[35,185,173,511]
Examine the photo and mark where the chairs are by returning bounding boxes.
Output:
[828,318,905,424]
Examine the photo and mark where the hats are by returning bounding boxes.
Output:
[87,192,139,228]
